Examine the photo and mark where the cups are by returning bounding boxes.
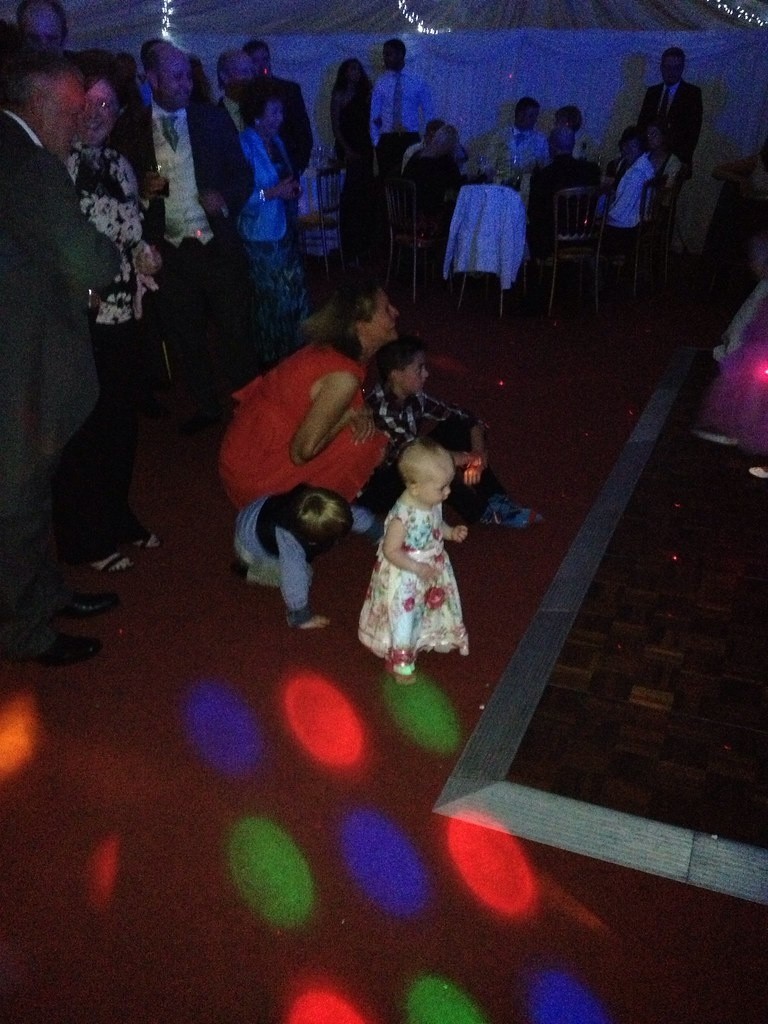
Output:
[151,164,169,196]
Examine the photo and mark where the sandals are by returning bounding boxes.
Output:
[132,531,162,548]
[91,551,134,574]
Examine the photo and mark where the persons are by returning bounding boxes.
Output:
[403,119,467,239]
[233,483,384,632]
[596,120,681,229]
[331,58,382,253]
[490,96,597,259]
[637,47,703,179]
[220,281,400,514]
[368,335,546,531]
[695,229,768,455]
[713,137,768,203]
[0,0,315,668]
[370,39,435,179]
[355,437,469,688]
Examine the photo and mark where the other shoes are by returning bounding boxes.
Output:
[749,467,768,479]
[480,493,543,528]
[391,667,417,686]
[713,345,726,363]
[688,421,739,446]
[360,521,385,549]
[133,387,167,418]
[178,405,225,439]
[229,558,249,579]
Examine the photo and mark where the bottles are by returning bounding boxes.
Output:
[579,142,588,160]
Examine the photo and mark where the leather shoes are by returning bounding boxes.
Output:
[58,590,120,618]
[31,632,102,665]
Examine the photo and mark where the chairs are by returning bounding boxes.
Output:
[292,162,690,321]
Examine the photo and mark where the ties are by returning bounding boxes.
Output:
[161,114,181,152]
[514,133,526,147]
[657,88,670,122]
[393,74,404,133]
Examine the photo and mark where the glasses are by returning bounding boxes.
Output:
[80,97,119,113]
[662,63,683,71]
